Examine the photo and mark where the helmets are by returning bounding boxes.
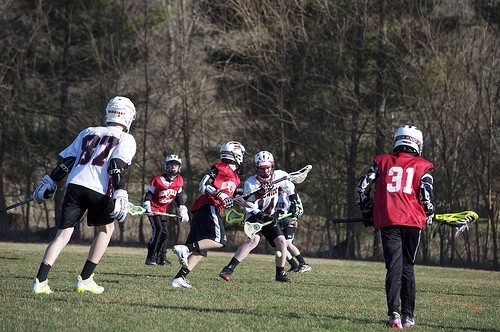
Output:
[164,154,182,175]
[106,96,136,133]
[393,125,423,157]
[254,150,275,178]
[220,140,249,168]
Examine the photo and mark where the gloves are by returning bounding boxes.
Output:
[143,201,151,214]
[177,205,189,222]
[361,208,375,228]
[425,212,435,225]
[287,206,297,218]
[33,174,58,204]
[215,191,233,208]
[257,212,279,227]
[109,189,129,223]
[254,184,269,200]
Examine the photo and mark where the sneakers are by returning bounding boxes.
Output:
[295,264,312,273]
[275,271,292,282]
[388,311,402,328]
[171,275,191,289]
[158,259,172,266]
[173,245,194,267]
[219,264,235,281]
[401,315,415,328]
[75,273,104,295]
[31,276,53,294]
[288,265,299,272]
[145,259,157,265]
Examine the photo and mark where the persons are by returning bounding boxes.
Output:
[276,186,312,274]
[30,96,137,294]
[355,125,436,330]
[219,151,293,283]
[171,140,269,289]
[143,153,190,266]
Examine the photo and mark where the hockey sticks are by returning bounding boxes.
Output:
[126,202,182,219]
[332,210,478,227]
[243,213,293,239]
[0,189,52,214]
[220,164,313,206]
[226,209,297,226]
